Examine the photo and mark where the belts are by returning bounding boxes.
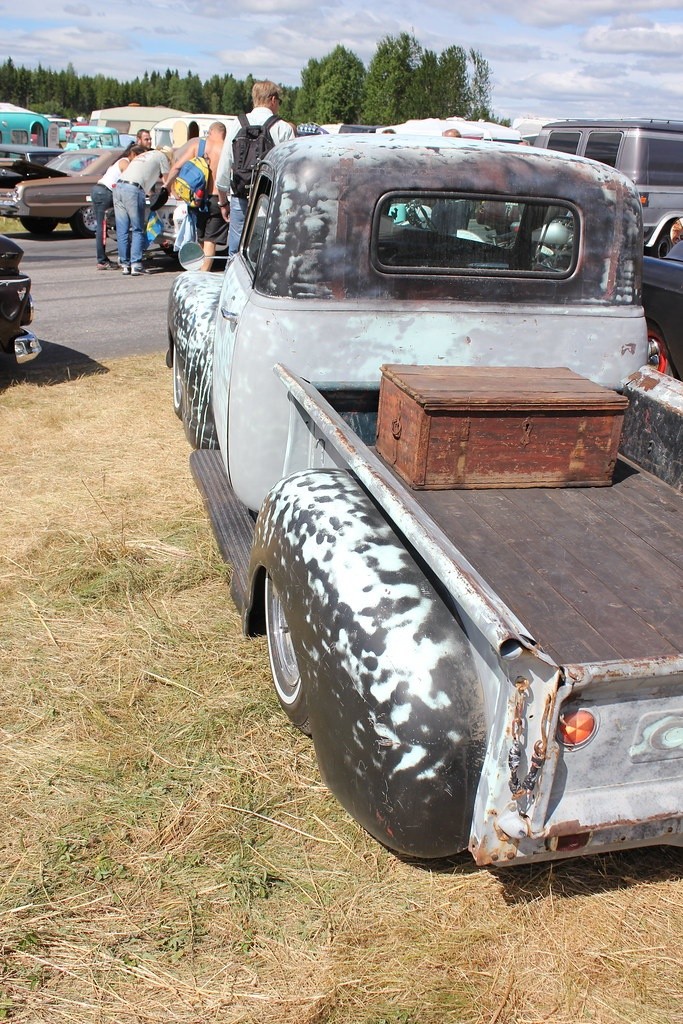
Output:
[117,179,143,190]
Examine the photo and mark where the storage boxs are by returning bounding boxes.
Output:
[376,363,630,491]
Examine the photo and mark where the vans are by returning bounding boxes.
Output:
[529,116,683,274]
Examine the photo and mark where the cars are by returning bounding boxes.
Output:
[0,226,42,366]
[639,241,683,383]
[0,144,129,239]
[0,108,123,165]
[0,143,67,177]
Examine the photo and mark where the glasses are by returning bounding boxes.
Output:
[276,97,282,107]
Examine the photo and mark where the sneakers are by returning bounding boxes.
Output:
[96,259,118,271]
[123,264,149,275]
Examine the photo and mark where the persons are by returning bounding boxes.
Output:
[111,145,172,275]
[441,128,471,230]
[215,81,296,272]
[160,121,230,271]
[100,129,155,197]
[289,121,298,138]
[90,145,145,271]
[519,143,528,146]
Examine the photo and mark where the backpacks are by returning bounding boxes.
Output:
[172,137,210,214]
[232,111,284,201]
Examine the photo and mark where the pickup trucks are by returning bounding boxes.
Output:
[167,129,681,873]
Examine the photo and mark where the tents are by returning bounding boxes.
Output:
[376,117,521,144]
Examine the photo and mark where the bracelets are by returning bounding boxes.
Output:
[217,199,230,207]
